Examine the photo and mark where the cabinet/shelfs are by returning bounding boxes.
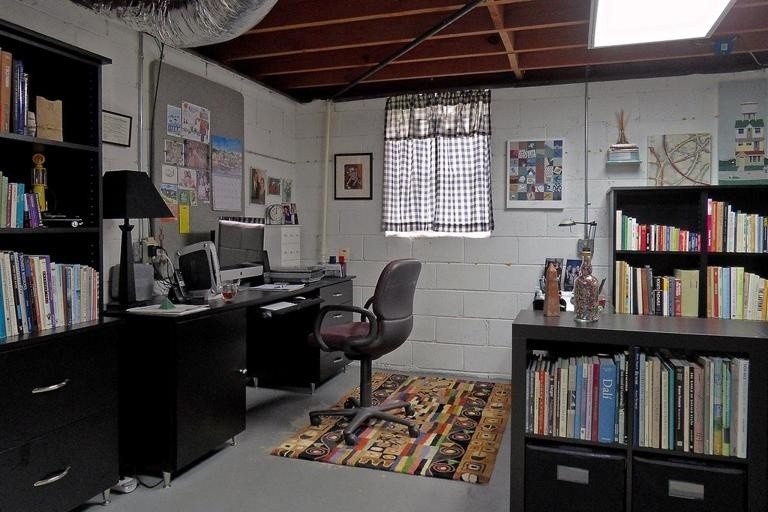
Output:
[264,224,302,267]
[0,20,125,511]
[609,185,768,321]
[510,311,768,512]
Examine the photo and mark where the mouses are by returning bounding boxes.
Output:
[294,296,307,300]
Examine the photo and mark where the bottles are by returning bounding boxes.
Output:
[574,251,599,324]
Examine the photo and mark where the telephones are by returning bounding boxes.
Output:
[168,264,187,301]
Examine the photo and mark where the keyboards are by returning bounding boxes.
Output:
[260,301,297,311]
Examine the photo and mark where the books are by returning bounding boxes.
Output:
[615,198,767,319]
[0,52,103,339]
[525,346,750,459]
[126,303,210,318]
[248,284,306,293]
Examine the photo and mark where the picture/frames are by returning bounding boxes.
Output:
[506,139,564,208]
[334,153,372,200]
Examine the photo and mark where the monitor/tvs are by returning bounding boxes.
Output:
[214,218,265,292]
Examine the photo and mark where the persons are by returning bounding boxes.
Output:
[565,263,581,291]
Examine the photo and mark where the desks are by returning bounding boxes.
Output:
[110,275,355,487]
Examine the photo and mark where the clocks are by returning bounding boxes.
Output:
[266,204,284,224]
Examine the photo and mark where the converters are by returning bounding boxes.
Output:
[148,245,158,256]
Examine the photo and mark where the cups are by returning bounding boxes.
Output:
[222,283,237,300]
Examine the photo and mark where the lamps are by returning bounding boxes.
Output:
[102,170,175,310]
[560,219,597,250]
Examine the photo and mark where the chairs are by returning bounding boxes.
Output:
[309,259,422,444]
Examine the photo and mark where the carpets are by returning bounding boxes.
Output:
[272,372,511,485]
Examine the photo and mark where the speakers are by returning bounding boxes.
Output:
[177,240,224,302]
[263,250,271,278]
